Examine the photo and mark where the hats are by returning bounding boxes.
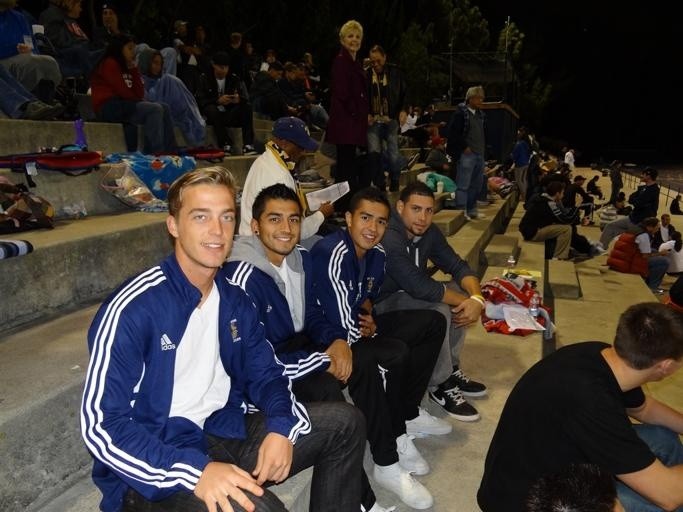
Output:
[574,176,587,182]
[431,135,445,145]
[642,167,659,177]
[271,115,320,151]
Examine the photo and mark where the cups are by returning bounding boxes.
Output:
[436,181,444,193]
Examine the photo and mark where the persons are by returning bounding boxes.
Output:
[252,20,403,201]
[78,117,489,512]
[398,84,683,308]
[0,1,266,160]
[476,297,682,512]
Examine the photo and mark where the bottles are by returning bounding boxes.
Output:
[73,119,87,149]
[507,256,516,272]
[529,295,538,320]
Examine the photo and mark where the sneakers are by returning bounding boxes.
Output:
[428,369,487,421]
[593,204,601,211]
[22,100,64,121]
[222,143,233,157]
[242,144,259,156]
[651,288,664,295]
[591,247,608,257]
[582,221,595,226]
[363,405,453,512]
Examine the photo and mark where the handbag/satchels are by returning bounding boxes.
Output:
[0,182,54,231]
